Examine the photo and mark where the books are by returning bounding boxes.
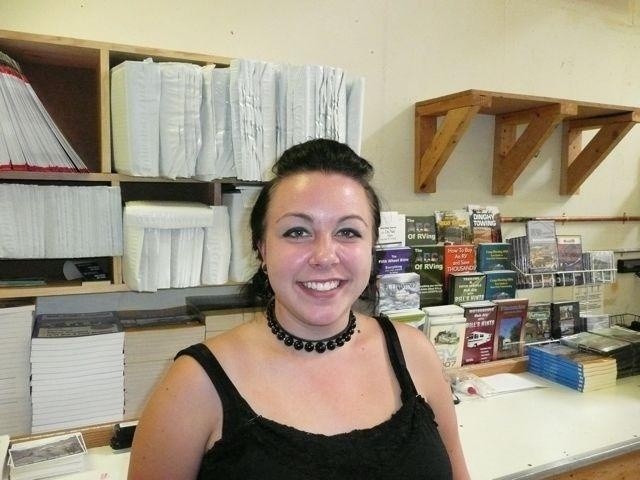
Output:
[507,220,558,289]
[0,269,56,288]
[422,305,467,369]
[381,308,425,333]
[433,207,472,245]
[0,49,92,173]
[449,271,486,305]
[460,301,498,366]
[373,244,412,274]
[476,242,511,271]
[74,261,112,286]
[412,246,445,307]
[527,342,618,394]
[490,298,529,362]
[552,298,580,340]
[374,271,421,318]
[184,293,268,341]
[588,325,640,376]
[8,432,87,480]
[374,211,406,250]
[581,250,617,283]
[404,215,438,247]
[524,301,552,356]
[0,299,38,439]
[31,310,125,436]
[561,330,634,379]
[116,305,206,421]
[554,235,584,285]
[1,434,11,480]
[444,245,477,292]
[484,270,518,301]
[465,205,502,244]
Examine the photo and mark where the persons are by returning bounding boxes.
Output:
[125,138,472,480]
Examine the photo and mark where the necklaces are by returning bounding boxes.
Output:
[266,300,358,353]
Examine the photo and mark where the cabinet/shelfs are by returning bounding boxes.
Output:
[1,28,368,303]
